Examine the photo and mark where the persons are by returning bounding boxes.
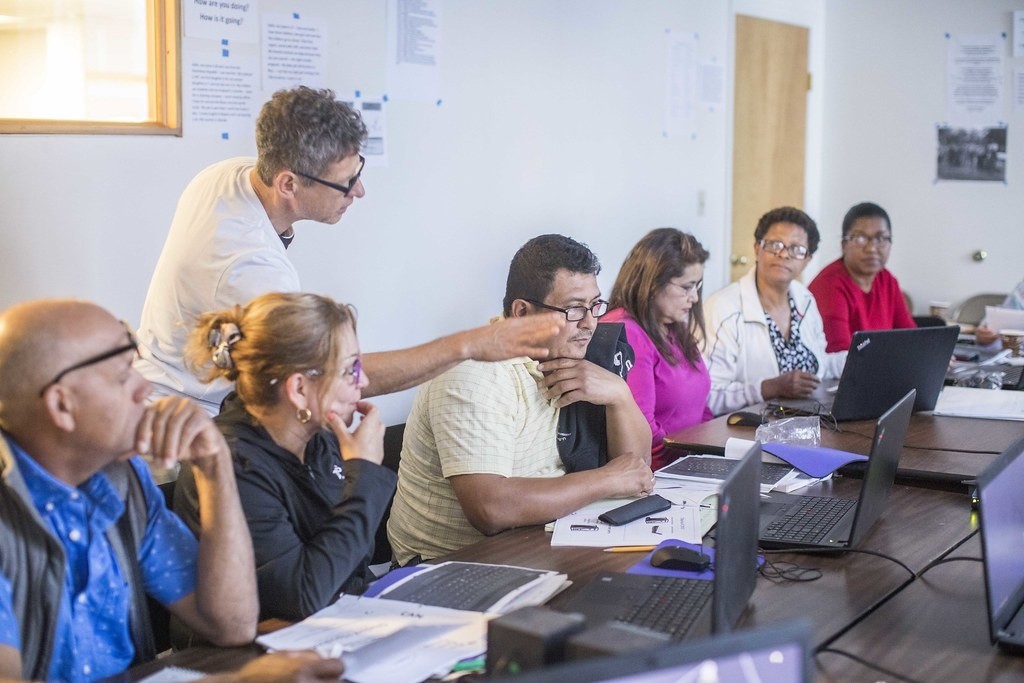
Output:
[385,234,656,576]
[807,201,957,381]
[592,228,711,474]
[1,298,346,683]
[691,206,849,416]
[124,84,566,522]
[167,292,401,645]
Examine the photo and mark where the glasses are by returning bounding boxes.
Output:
[662,279,703,297]
[270,361,361,389]
[40,320,144,398]
[291,153,365,198]
[842,231,892,248]
[757,238,811,261]
[523,298,610,322]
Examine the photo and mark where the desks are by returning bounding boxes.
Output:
[89,477,1024,683]
[662,401,1024,482]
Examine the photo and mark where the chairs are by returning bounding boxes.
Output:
[951,293,1009,327]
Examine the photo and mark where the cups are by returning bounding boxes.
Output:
[999,329,1024,358]
[757,416,821,447]
[928,302,948,318]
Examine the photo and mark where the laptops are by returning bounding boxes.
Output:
[708,389,917,557]
[771,325,961,424]
[560,440,761,656]
[975,436,1024,658]
[487,620,815,683]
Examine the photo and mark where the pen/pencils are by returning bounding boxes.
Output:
[602,545,657,553]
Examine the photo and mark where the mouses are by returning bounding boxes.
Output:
[650,545,711,571]
[728,412,769,427]
